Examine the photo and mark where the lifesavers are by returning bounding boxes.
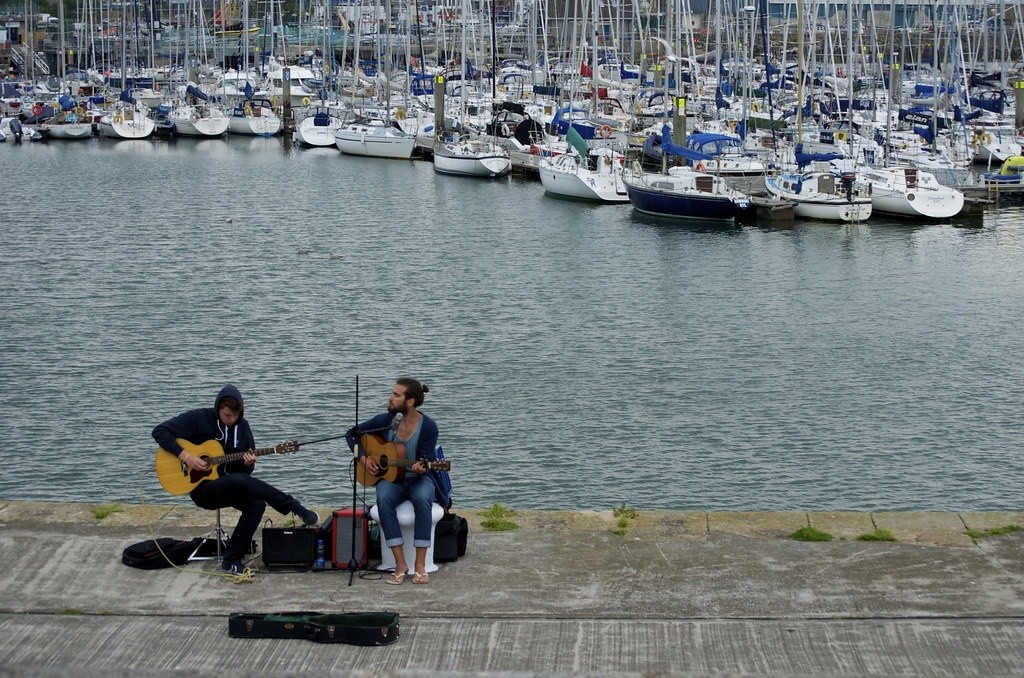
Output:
[599,124,612,138]
[979,132,992,145]
[113,114,123,124]
[244,105,250,114]
[498,85,505,93]
[835,130,847,141]
[32,105,43,115]
[752,102,760,111]
[396,109,406,120]
[301,96,310,105]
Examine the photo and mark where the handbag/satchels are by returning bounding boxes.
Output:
[433,514,468,564]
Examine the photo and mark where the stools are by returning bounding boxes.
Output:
[371,502,445,576]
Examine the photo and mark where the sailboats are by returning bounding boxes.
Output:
[1,0,1024,230]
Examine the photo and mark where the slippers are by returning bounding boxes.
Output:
[412,572,429,584]
[386,572,408,584]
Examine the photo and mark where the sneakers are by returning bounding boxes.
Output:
[221,558,255,576]
[291,500,320,526]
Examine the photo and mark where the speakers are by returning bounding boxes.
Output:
[262,525,318,566]
[332,509,367,567]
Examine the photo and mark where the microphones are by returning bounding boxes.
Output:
[390,413,403,443]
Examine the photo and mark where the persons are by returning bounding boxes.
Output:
[152,384,320,578]
[346,378,452,586]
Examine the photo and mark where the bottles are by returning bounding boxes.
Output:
[316,540,325,569]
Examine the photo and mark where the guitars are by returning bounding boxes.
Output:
[154,434,300,497]
[353,433,451,488]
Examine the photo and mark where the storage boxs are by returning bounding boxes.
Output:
[332,506,368,571]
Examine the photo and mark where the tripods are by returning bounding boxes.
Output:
[298,377,394,585]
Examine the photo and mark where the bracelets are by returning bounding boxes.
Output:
[181,452,189,463]
